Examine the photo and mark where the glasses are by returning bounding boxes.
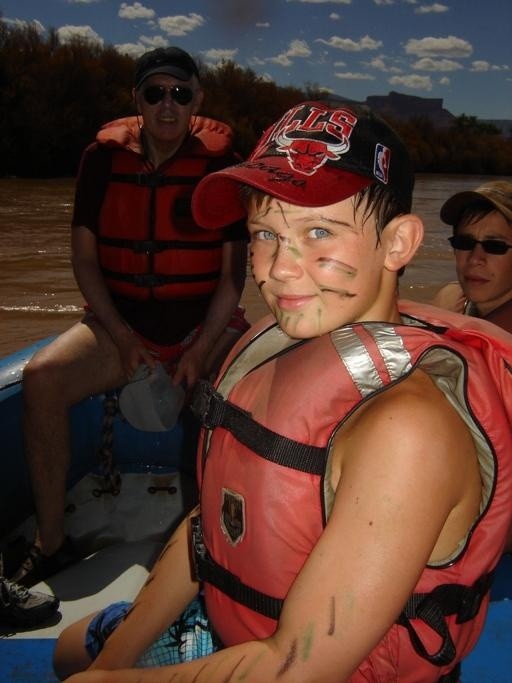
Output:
[140,85,194,106]
[447,235,512,254]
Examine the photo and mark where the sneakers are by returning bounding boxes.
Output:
[0,528,72,626]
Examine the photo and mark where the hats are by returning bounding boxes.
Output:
[438,181,512,223]
[192,100,415,230]
[134,46,199,89]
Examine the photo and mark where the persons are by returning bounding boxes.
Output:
[53,100,482,683]
[12,49,251,585]
[433,180,512,335]
